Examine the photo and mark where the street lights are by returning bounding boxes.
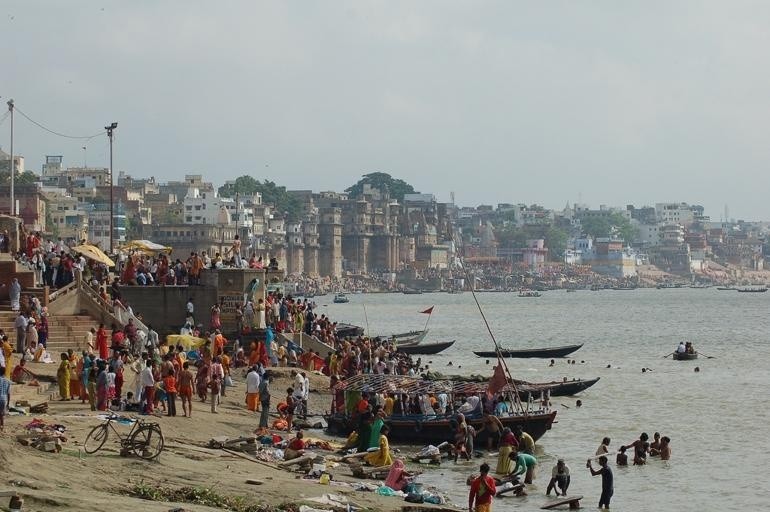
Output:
[105,122,117,252]
[7,99,15,217]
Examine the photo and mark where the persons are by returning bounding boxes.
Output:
[677,341,695,354]
[2,231,508,510]
[498,360,671,511]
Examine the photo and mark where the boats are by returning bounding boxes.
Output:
[613,287,635,290]
[473,343,584,359]
[323,373,557,450]
[518,293,541,297]
[661,283,768,292]
[423,369,600,402]
[334,293,349,303]
[334,320,363,338]
[673,350,699,360]
[372,329,456,354]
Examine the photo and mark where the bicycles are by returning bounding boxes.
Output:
[85,405,164,459]
